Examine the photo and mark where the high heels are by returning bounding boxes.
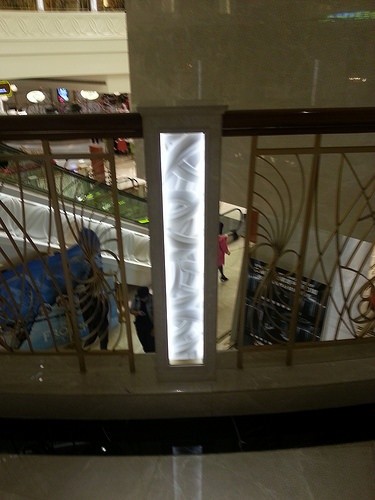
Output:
[221,276,228,281]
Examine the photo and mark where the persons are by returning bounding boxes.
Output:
[77,282,110,351]
[128,286,156,353]
[216,221,231,281]
